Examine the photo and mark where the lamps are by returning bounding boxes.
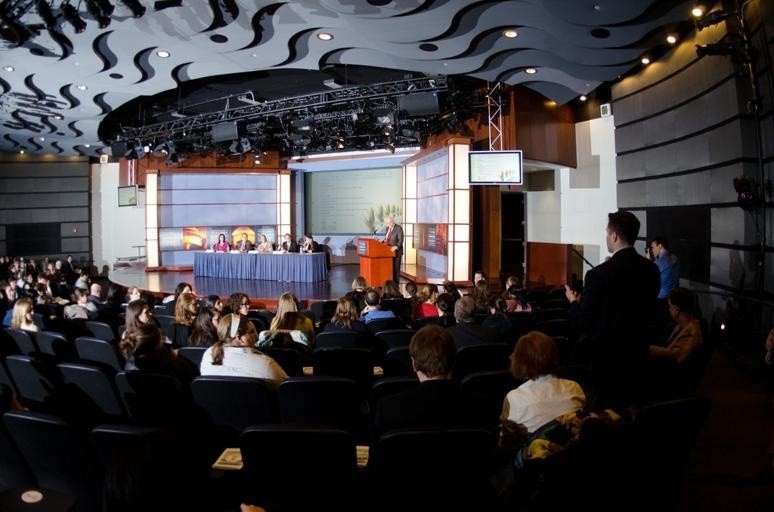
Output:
[97,76,447,167]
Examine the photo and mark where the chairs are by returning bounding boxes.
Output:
[0,256,773,512]
[212,243,329,268]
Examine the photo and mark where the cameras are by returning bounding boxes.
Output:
[645,247,653,255]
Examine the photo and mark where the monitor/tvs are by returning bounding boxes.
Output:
[468,150,523,185]
[118,186,138,206]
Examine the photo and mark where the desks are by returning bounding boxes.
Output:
[193,251,328,285]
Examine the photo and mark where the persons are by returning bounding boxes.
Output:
[765,328,774,365]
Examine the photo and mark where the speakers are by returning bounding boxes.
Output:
[396,90,439,117]
[211,120,238,144]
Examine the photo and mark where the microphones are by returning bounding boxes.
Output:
[371,229,378,240]
